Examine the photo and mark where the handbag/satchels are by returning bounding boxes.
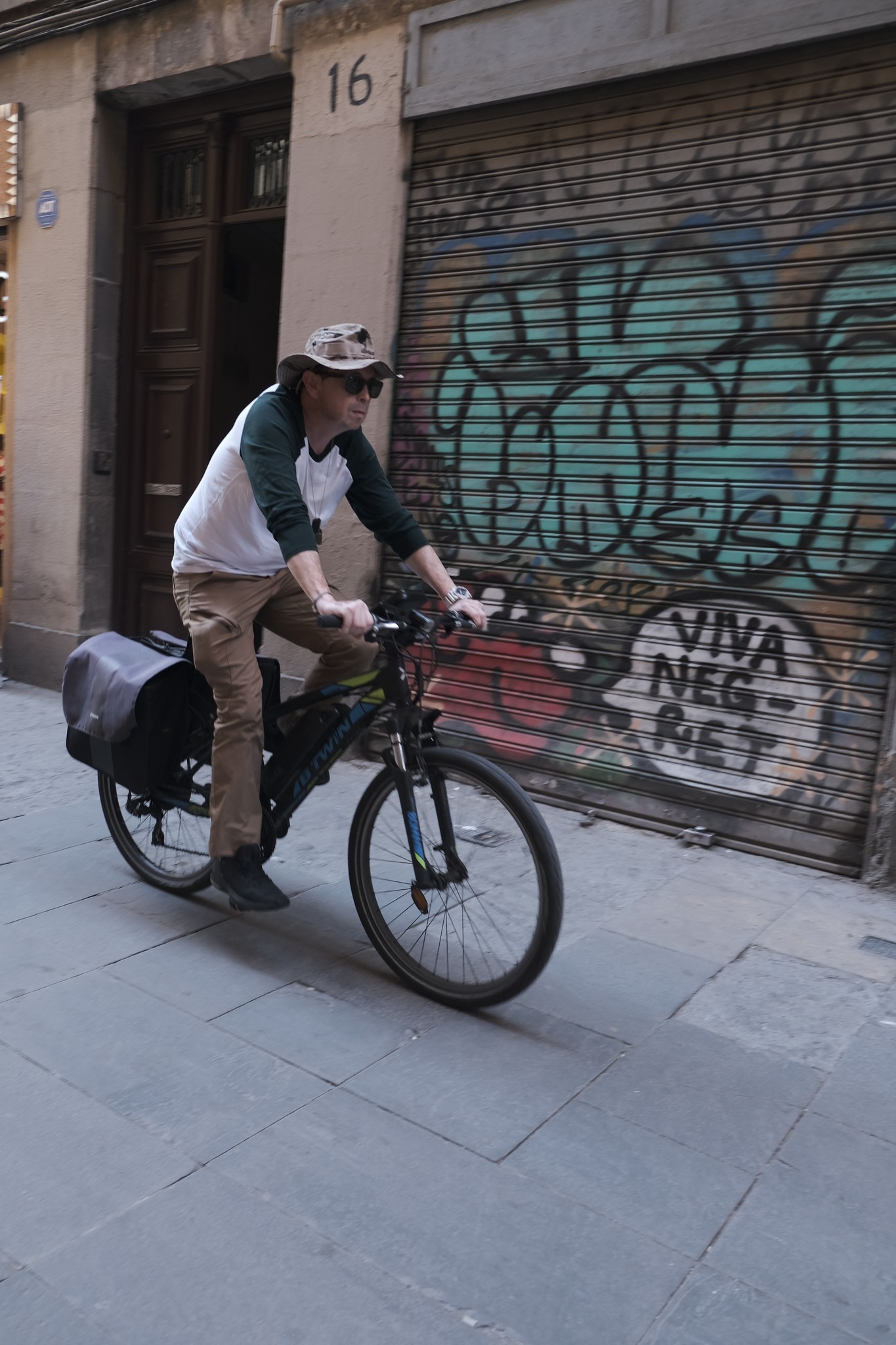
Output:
[61,631,218,800]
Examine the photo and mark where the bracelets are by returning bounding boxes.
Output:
[311,592,332,614]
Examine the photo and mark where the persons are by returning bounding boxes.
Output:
[171,322,488,910]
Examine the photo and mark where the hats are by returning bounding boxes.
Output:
[276,323,404,395]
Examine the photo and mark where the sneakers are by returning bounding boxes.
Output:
[209,844,290,909]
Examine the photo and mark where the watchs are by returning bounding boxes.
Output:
[443,586,473,609]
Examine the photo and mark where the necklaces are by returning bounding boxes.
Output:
[309,439,334,546]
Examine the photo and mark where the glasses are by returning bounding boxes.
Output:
[320,373,384,399]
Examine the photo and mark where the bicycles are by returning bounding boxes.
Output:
[61,588,566,1014]
